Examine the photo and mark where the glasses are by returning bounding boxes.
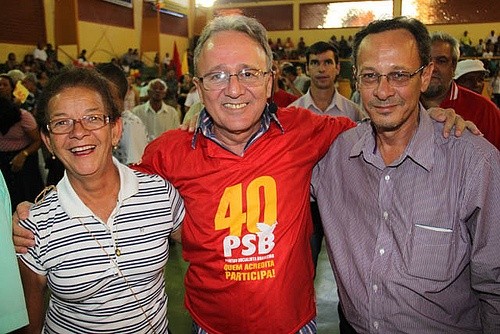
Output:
[198,68,268,90]
[356,65,424,87]
[45,113,109,135]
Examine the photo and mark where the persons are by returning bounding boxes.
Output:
[0,15,500,334]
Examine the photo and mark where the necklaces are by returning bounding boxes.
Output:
[66,170,120,256]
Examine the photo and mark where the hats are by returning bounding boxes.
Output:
[454,59,490,79]
